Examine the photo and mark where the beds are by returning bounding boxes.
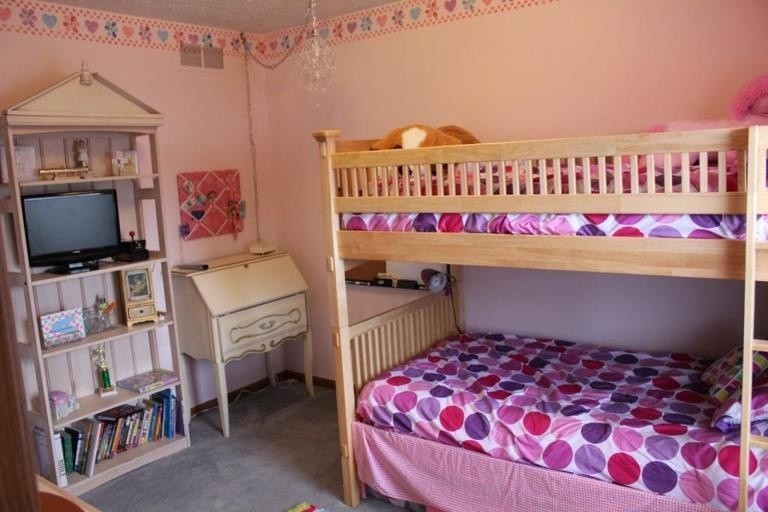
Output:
[312,128,767,512]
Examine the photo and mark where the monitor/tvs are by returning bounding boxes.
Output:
[21,189,123,276]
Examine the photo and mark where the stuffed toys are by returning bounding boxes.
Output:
[369,124,481,179]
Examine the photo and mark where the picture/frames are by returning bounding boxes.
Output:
[120,267,158,329]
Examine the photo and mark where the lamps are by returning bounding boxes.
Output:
[299,1,333,111]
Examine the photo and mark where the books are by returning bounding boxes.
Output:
[53,388,186,489]
[116,368,179,395]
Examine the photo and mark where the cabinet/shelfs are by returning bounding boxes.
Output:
[170,250,310,442]
[0,61,191,499]
